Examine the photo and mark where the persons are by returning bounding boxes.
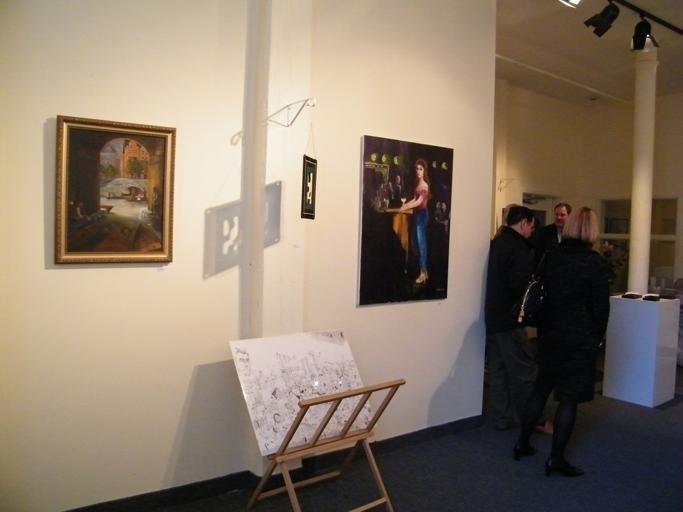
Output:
[364,170,451,236]
[482,205,552,436]
[511,204,611,478]
[526,200,572,251]
[398,156,432,283]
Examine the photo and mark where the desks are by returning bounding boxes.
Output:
[603,293,681,408]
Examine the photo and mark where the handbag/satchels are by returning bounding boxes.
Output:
[516,279,543,326]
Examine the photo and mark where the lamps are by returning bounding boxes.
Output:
[560,0,683,51]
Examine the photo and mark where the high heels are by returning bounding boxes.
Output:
[512,441,584,478]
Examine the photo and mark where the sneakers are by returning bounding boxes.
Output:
[536,419,553,435]
[415,271,429,284]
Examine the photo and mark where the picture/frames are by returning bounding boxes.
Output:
[54,113,176,264]
